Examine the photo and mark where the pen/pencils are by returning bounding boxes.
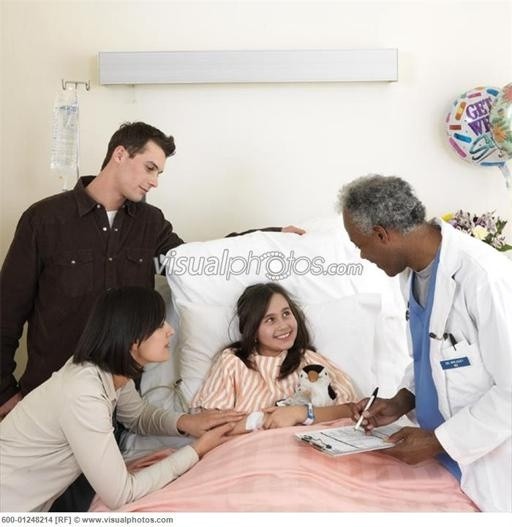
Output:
[353,387,378,427]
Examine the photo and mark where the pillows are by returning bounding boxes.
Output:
[176,296,385,403]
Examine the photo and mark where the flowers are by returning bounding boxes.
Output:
[438,207,512,256]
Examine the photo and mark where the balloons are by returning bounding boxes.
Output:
[445,85,512,173]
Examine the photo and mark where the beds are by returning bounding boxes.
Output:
[90,230,479,511]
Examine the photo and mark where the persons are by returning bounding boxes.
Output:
[0,120,306,512]
[0,283,246,512]
[190,280,362,435]
[338,175,512,513]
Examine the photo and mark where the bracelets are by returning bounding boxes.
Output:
[302,400,316,426]
